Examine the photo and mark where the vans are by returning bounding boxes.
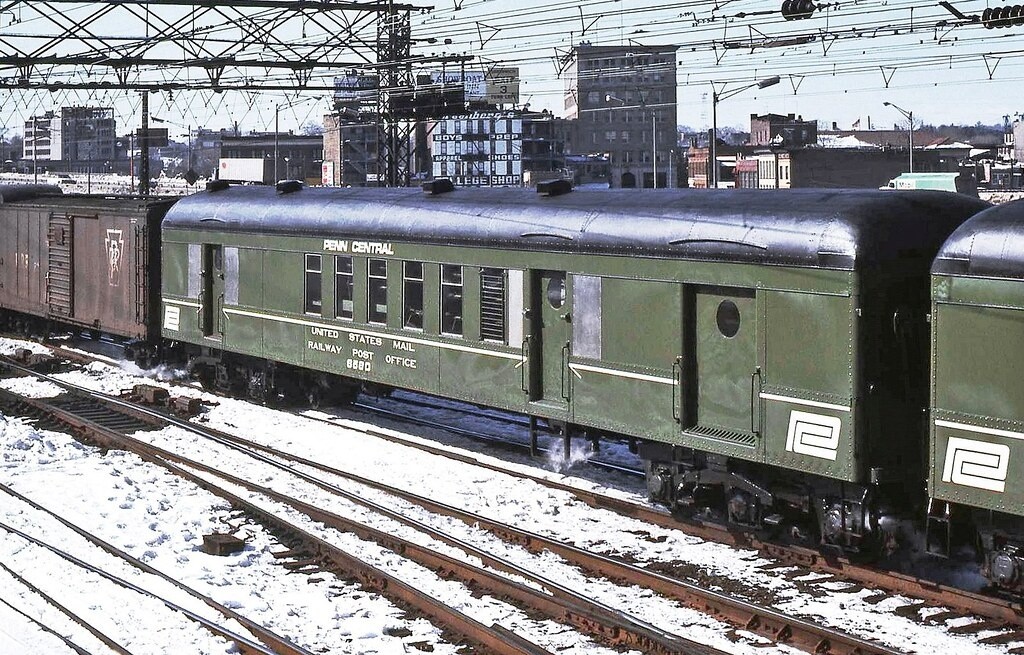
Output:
[888,171,979,199]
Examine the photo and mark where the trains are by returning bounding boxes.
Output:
[0,167,1024,606]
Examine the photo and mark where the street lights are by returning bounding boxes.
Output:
[275,94,322,185]
[883,101,913,173]
[712,77,781,187]
